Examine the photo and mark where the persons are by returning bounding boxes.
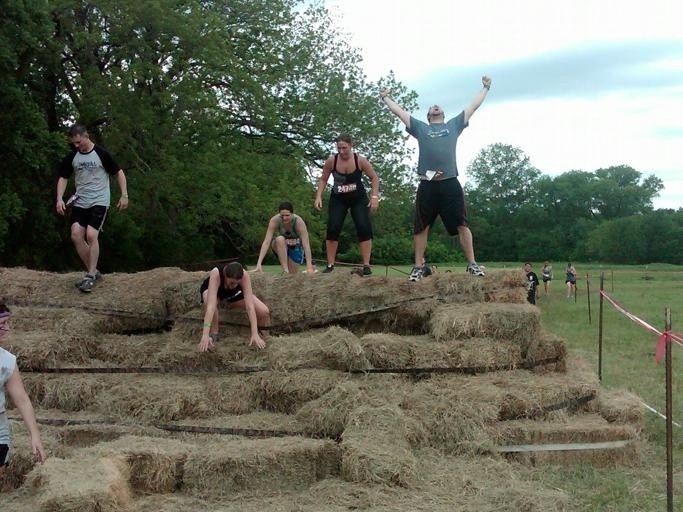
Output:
[523,262,541,305]
[430,264,437,274]
[313,132,380,276]
[541,260,553,297]
[0,300,47,481]
[197,261,269,353]
[378,73,492,284]
[564,262,576,298]
[54,123,130,293]
[246,201,316,276]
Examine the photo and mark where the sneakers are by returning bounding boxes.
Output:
[363,265,371,275]
[79,277,95,292]
[324,265,333,273]
[408,266,431,281]
[466,263,484,276]
[86,270,101,282]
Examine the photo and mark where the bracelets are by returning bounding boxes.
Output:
[482,84,489,90]
[380,94,387,101]
[203,323,212,329]
[120,193,128,199]
[370,194,378,199]
